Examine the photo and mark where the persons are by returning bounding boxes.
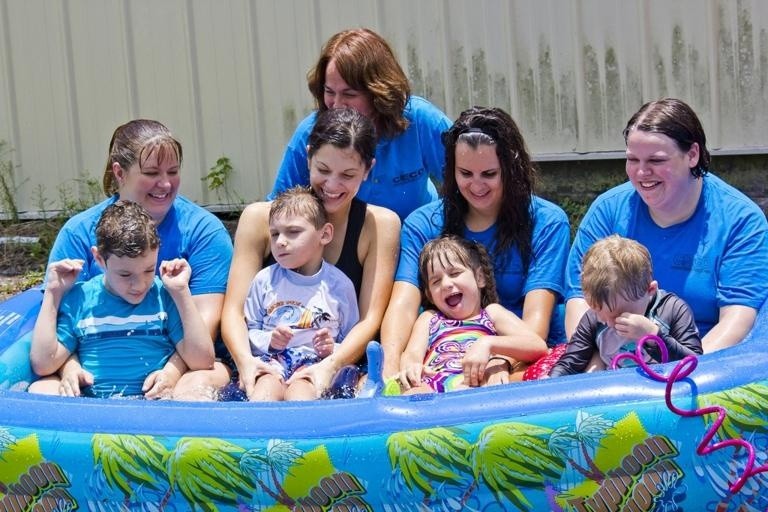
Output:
[219,106,401,398]
[537,234,704,378]
[376,103,572,379]
[243,183,362,402]
[398,232,548,396]
[560,96,768,370]
[28,119,235,406]
[266,24,460,223]
[29,192,216,400]
